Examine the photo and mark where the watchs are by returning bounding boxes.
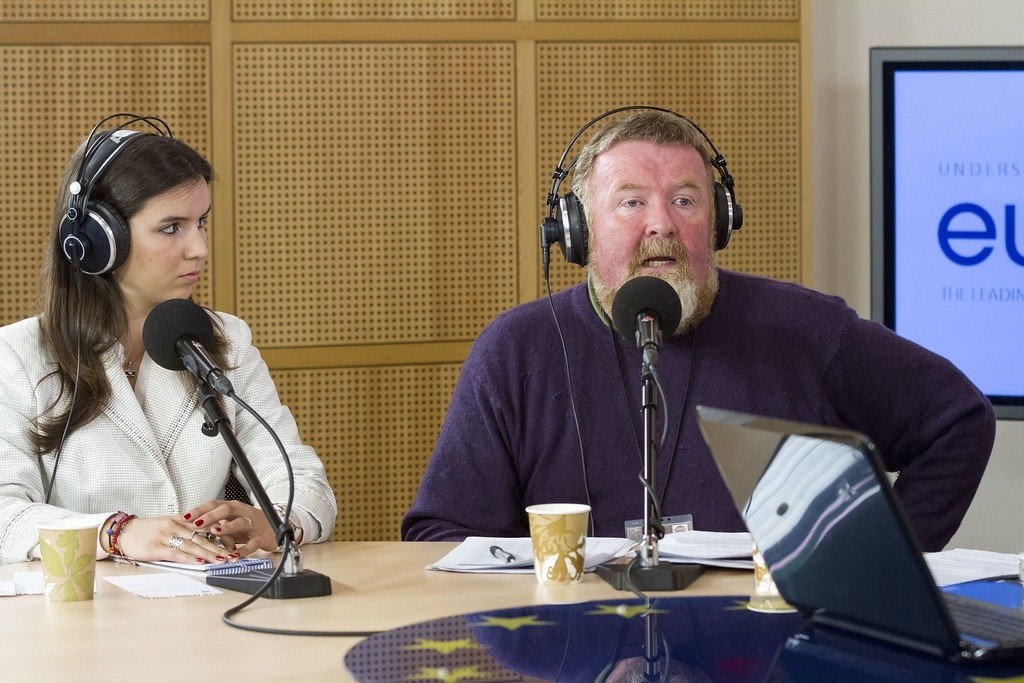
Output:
[273,503,302,542]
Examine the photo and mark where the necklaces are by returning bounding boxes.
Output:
[123,354,142,377]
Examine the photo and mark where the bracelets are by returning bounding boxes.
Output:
[106,511,139,566]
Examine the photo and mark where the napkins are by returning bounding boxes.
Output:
[15,570,97,596]
[102,572,223,599]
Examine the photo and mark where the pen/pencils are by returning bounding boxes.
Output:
[195,531,223,544]
[489,546,516,562]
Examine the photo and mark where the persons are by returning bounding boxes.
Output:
[0,131,338,564]
[400,112,997,553]
[674,526,686,533]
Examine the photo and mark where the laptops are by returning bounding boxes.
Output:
[758,625,1024,683]
[693,405,1023,663]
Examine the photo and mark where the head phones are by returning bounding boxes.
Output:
[539,106,743,268]
[58,112,173,276]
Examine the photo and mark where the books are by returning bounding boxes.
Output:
[426,530,753,575]
[150,558,270,571]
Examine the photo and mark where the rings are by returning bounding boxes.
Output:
[170,536,185,550]
[242,515,254,530]
[189,531,199,540]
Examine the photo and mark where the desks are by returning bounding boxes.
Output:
[0,537,1024,683]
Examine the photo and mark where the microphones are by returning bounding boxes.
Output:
[612,276,682,367]
[142,297,234,398]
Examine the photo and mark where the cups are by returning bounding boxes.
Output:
[525,503,592,584]
[746,537,800,613]
[38,520,101,601]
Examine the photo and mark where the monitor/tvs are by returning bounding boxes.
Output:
[868,43,1024,421]
